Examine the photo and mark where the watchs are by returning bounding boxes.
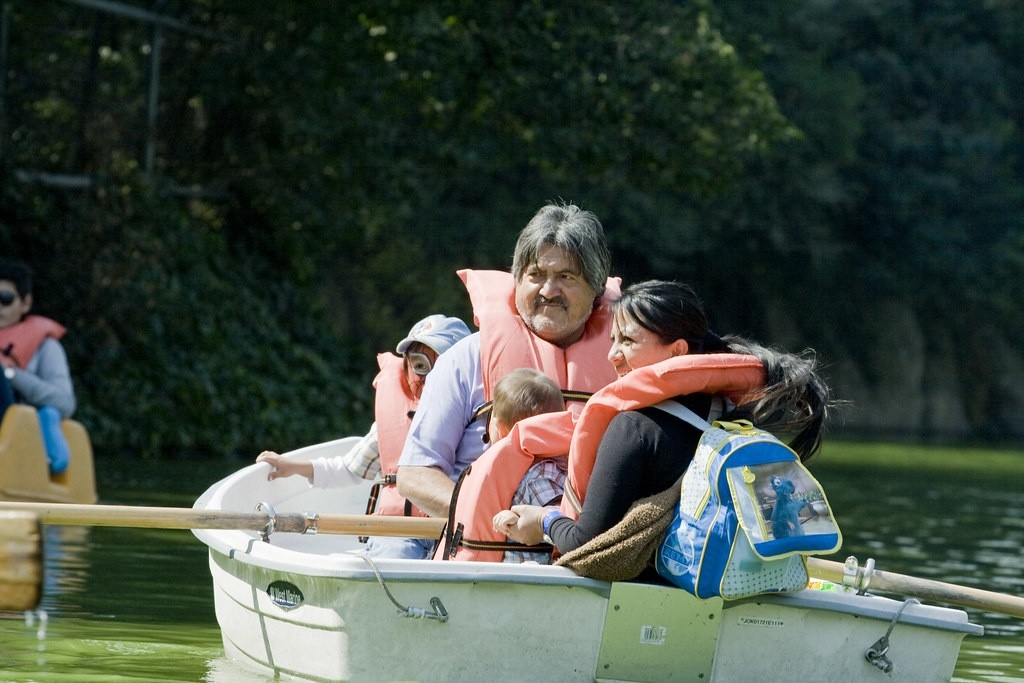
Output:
[4,365,15,382]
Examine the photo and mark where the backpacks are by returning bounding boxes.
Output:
[648,394,843,601]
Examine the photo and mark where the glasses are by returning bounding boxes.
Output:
[0,291,22,307]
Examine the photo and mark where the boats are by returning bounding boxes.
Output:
[1,402,99,506]
[188,435,968,683]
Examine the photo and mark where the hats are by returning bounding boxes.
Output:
[396,314,472,358]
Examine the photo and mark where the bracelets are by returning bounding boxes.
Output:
[543,511,563,534]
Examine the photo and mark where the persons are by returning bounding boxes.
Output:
[256,205,830,586]
[0,259,74,473]
